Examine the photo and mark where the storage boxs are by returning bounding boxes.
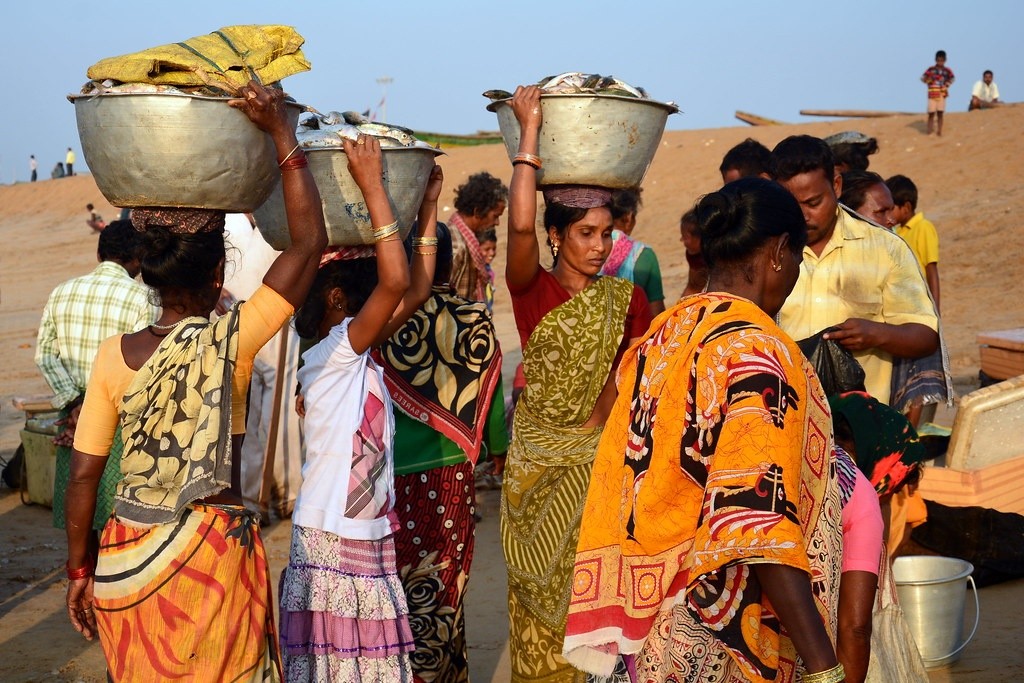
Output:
[976,325,1024,379]
[917,373,1024,515]
[18,417,58,507]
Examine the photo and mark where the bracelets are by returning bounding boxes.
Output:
[685,249,708,269]
[65,559,97,580]
[277,144,309,171]
[410,234,438,255]
[372,219,403,241]
[801,663,847,683]
[511,152,542,170]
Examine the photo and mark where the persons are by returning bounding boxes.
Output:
[502,86,657,683]
[968,70,1004,112]
[595,187,666,315]
[295,220,509,683]
[444,171,509,319]
[719,131,953,405]
[920,50,956,136]
[65,147,75,177]
[217,212,307,525]
[51,162,65,179]
[30,155,38,182]
[281,132,444,683]
[64,78,328,682]
[33,219,164,569]
[567,178,850,683]
[85,204,106,234]
[827,390,925,683]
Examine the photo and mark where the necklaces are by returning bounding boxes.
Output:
[150,315,196,330]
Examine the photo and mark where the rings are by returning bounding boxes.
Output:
[356,139,366,146]
[85,608,93,614]
[247,91,257,101]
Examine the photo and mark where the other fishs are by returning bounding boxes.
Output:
[482,71,686,115]
[296,110,449,157]
[86,81,190,103]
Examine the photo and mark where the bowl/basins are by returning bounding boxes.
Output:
[67,93,308,215]
[486,92,680,191]
[251,145,445,251]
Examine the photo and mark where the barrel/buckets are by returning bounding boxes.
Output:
[892,555,980,669]
